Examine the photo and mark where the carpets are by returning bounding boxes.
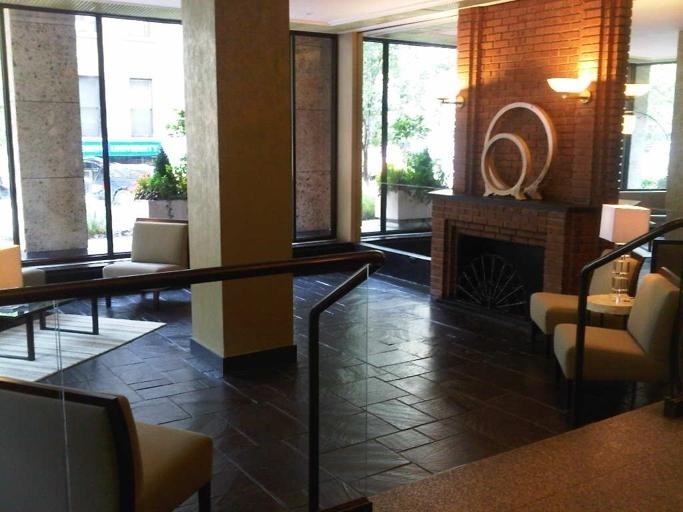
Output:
[0,314,168,383]
[362,398,683,512]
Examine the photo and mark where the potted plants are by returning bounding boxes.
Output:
[131,146,188,220]
[374,146,448,221]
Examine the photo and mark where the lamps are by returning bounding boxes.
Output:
[433,80,465,108]
[621,110,639,136]
[599,203,651,303]
[624,83,648,102]
[547,77,592,103]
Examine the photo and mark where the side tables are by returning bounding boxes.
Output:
[586,293,634,330]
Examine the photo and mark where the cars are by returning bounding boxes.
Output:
[0,149,151,215]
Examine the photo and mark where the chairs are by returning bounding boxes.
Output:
[102,217,189,310]
[529,246,646,360]
[1,376,214,512]
[552,265,683,428]
